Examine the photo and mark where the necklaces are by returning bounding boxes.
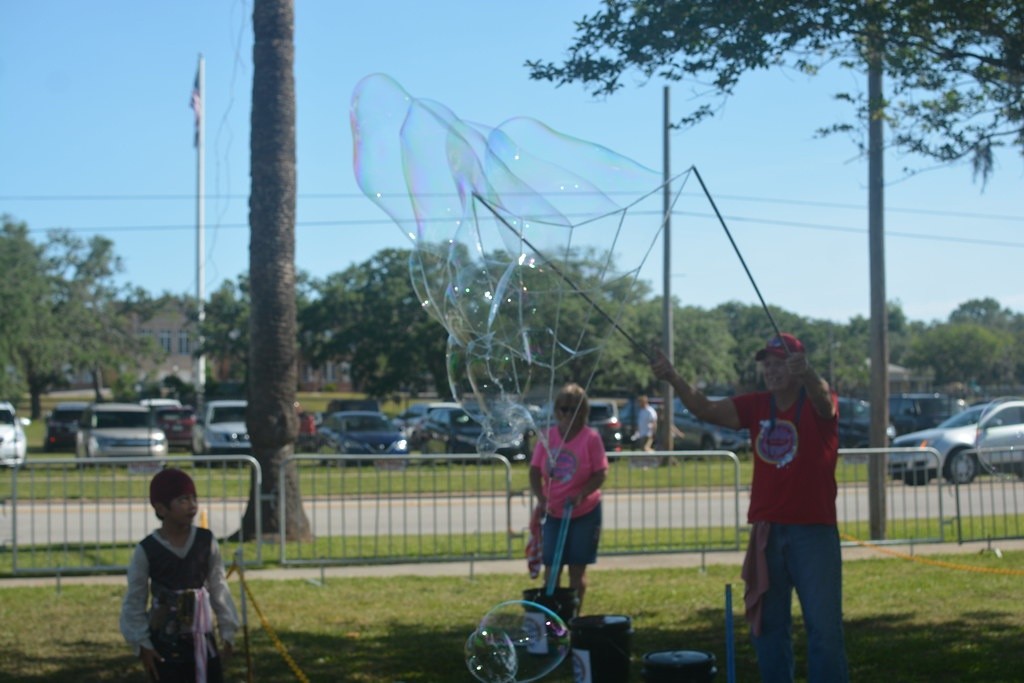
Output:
[766,387,804,468]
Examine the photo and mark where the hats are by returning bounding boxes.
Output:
[149,468,196,508]
[755,333,807,359]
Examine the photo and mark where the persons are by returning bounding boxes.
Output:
[631,396,684,468]
[529,382,608,617]
[650,334,842,683]
[120,469,239,683]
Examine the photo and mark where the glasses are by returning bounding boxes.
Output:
[558,406,581,414]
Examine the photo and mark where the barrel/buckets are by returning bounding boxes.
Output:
[522,588,580,655]
[568,618,635,683]
[642,650,719,683]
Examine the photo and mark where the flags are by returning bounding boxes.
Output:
[189,69,200,149]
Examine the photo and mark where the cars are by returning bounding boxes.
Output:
[151,405,198,450]
[536,398,623,463]
[0,401,32,471]
[392,401,533,465]
[43,402,95,451]
[833,393,969,449]
[316,410,409,468]
[320,398,379,421]
[622,391,753,452]
[75,404,168,467]
[890,397,1024,485]
[194,400,253,468]
